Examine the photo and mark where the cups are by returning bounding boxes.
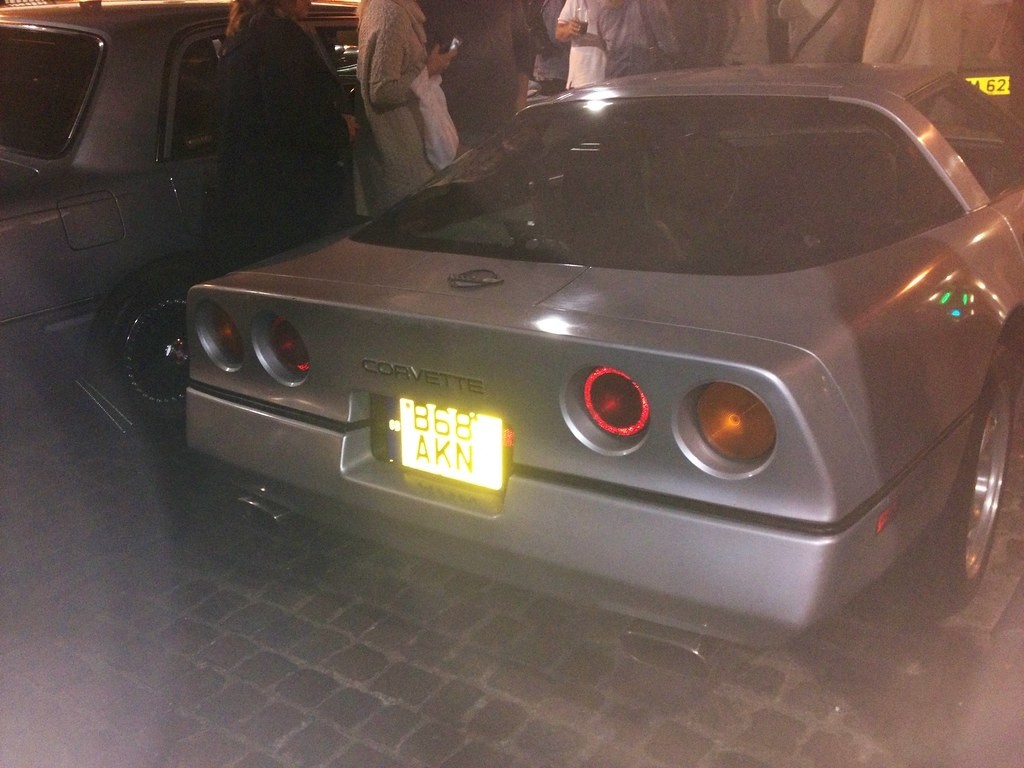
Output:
[576,9,588,34]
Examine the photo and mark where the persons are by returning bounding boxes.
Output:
[356,0,464,223]
[526,0,861,104]
[217,0,361,275]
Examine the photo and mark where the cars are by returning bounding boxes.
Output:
[179,58,1024,678]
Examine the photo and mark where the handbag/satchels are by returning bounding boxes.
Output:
[410,66,459,168]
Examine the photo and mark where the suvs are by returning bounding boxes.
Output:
[0,0,356,444]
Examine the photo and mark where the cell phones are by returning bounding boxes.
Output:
[447,34,462,52]
